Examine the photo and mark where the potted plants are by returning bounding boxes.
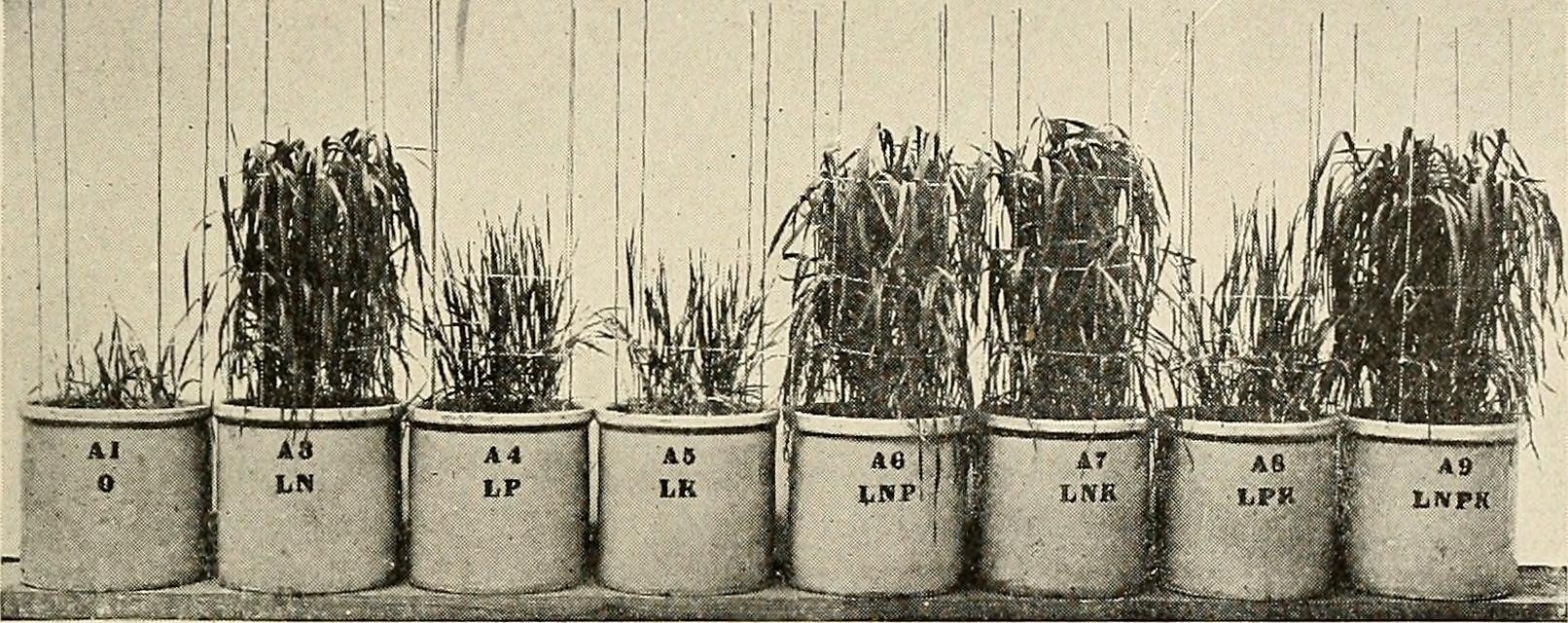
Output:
[598,234,778,590]
[212,130,420,595]
[408,226,594,594]
[1158,180,1342,598]
[783,127,973,595]
[16,311,210,591]
[978,124,1155,597]
[1343,137,1568,601]
